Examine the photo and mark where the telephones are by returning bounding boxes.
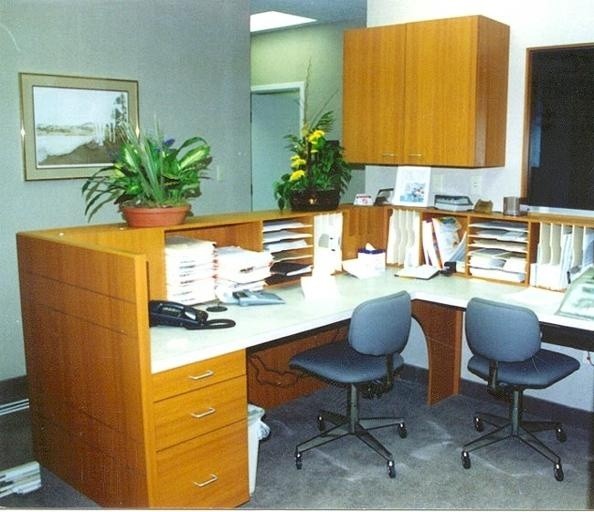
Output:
[149,301,207,328]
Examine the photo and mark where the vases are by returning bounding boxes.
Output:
[289,190,340,212]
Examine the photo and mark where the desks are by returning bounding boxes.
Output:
[16,204,594,508]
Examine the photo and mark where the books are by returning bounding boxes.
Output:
[155,203,594,319]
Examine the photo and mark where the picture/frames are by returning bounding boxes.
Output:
[17,71,140,181]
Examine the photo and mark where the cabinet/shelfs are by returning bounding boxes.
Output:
[343,14,509,169]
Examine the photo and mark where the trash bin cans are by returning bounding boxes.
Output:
[248,403,266,497]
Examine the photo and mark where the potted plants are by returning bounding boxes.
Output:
[81,128,212,230]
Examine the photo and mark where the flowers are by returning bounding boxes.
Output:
[271,111,351,196]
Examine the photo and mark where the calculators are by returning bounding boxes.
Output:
[232,290,286,304]
